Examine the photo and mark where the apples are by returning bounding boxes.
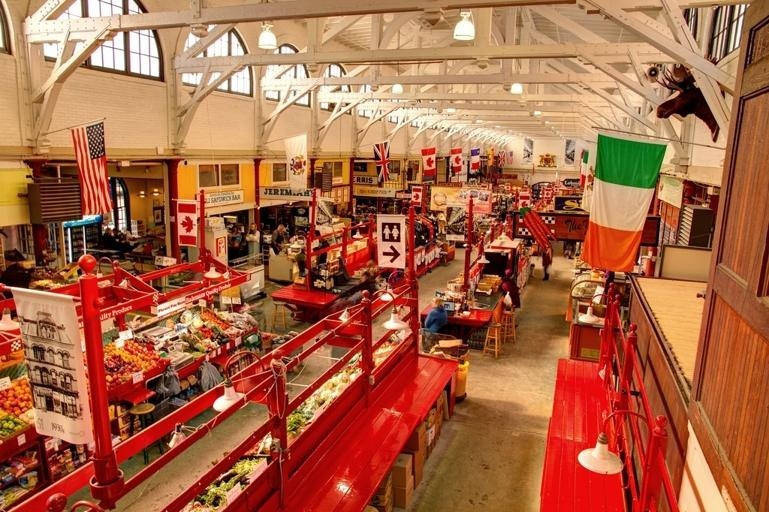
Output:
[0,379,33,437]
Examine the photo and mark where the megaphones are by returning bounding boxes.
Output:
[647,67,659,83]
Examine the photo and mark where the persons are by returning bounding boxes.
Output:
[354,271,378,295]
[314,229,329,266]
[245,223,261,255]
[271,224,288,255]
[542,244,553,281]
[1,253,27,319]
[102,228,118,252]
[424,297,448,334]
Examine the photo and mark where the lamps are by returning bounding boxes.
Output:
[258,21,277,50]
[453,8,476,41]
[391,19,404,95]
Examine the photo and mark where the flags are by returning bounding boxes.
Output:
[470,148,481,174]
[485,143,494,166]
[576,133,666,273]
[498,151,506,169]
[372,141,390,183]
[450,147,462,172]
[71,119,113,217]
[421,147,437,176]
[285,133,308,194]
[507,151,513,164]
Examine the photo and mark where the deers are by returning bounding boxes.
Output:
[655,63,718,134]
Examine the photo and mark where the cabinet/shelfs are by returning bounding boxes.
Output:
[537,250,681,508]
[2,186,544,508]
